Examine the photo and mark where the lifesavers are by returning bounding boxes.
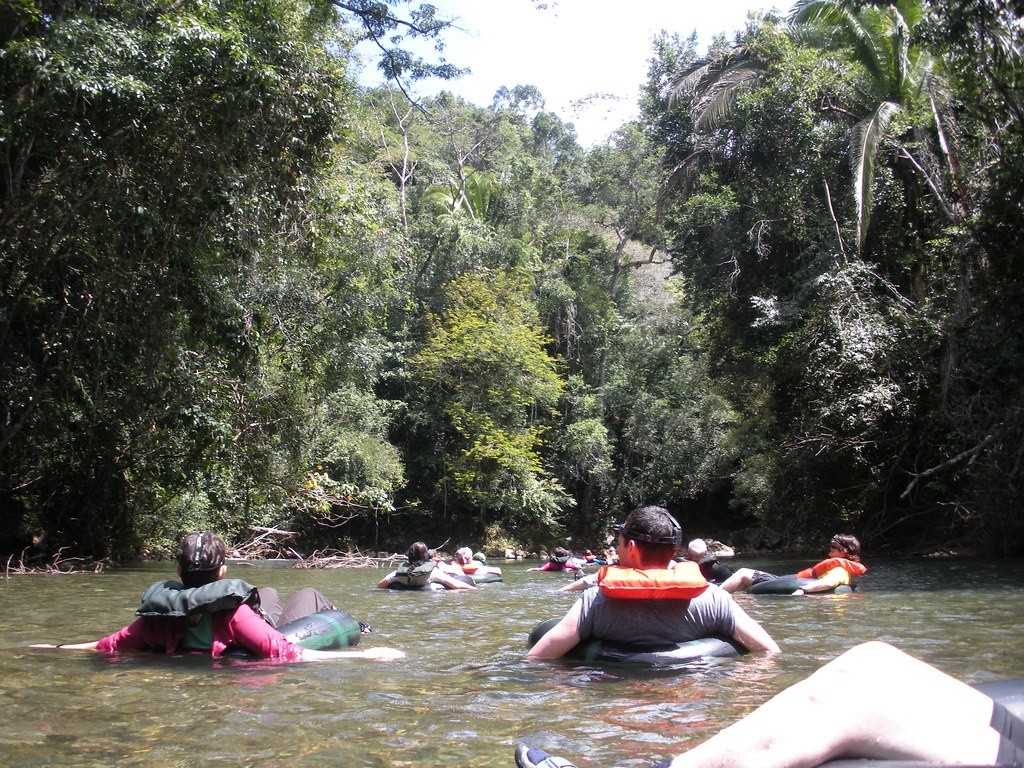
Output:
[415,573,480,594]
[739,573,863,601]
[213,602,369,665]
[462,570,507,585]
[810,667,1024,768]
[538,557,621,573]
[521,612,744,683]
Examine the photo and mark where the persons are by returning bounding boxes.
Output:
[29,530,404,665]
[378,506,866,659]
[514,640,1024,768]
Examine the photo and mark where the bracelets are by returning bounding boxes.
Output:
[56,644,64,648]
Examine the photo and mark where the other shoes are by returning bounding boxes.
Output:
[514,743,579,768]
[358,622,372,635]
